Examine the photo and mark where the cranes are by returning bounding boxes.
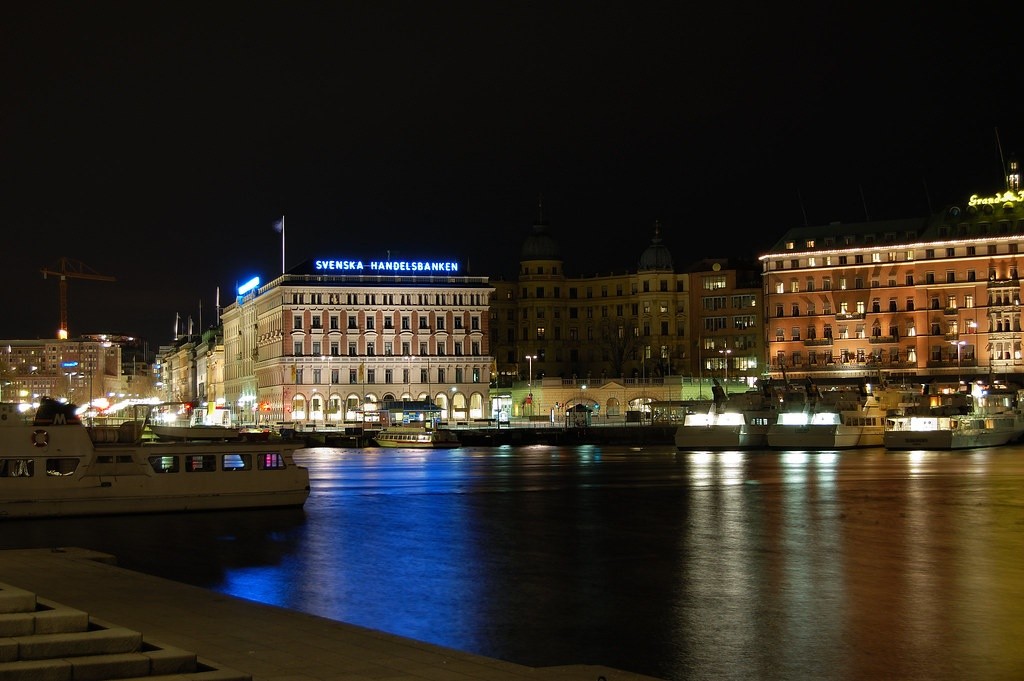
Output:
[41,255,118,341]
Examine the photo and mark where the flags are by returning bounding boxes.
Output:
[275,219,283,231]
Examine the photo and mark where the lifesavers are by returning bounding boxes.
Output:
[31,429,49,448]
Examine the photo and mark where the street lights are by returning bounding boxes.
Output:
[580,384,587,406]
[956,340,969,397]
[524,354,537,417]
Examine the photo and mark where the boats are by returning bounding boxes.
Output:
[673,362,1024,451]
[371,425,461,449]
[0,401,311,520]
